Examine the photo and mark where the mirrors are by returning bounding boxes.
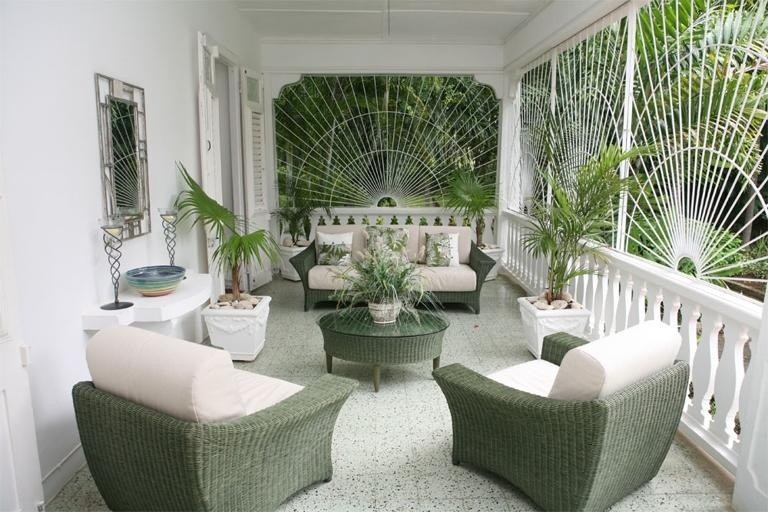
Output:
[95,73,152,244]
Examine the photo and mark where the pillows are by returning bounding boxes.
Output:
[316,225,366,266]
[425,232,460,266]
[385,225,419,263]
[315,231,353,266]
[548,320,682,403]
[85,325,244,424]
[419,225,471,264]
[363,226,409,266]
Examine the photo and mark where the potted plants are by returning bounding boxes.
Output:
[174,160,284,361]
[329,237,445,326]
[442,166,505,282]
[269,199,331,281]
[517,142,661,360]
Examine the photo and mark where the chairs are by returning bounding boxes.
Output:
[72,326,359,512]
[431,321,690,512]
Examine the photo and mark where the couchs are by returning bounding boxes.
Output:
[290,224,496,314]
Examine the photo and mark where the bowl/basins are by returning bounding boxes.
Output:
[125,264,186,299]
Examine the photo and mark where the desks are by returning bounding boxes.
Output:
[81,272,213,343]
